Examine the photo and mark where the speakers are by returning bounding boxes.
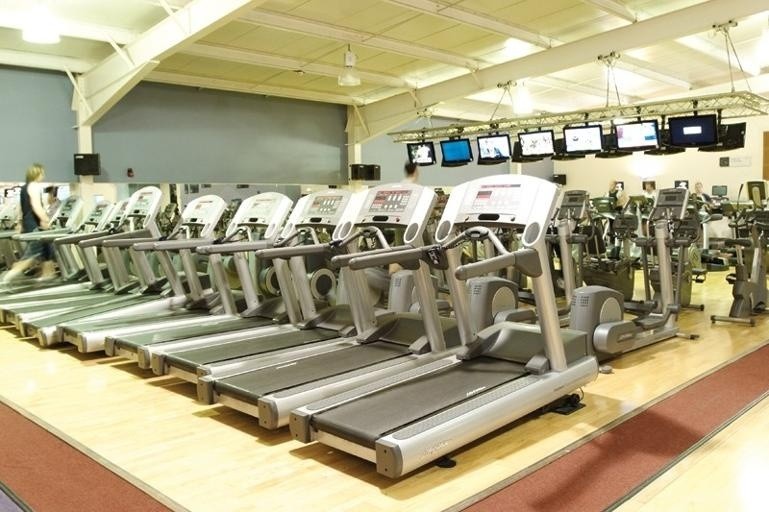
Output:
[367,164,381,179]
[351,164,367,180]
[74,154,101,175]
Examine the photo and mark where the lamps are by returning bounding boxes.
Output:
[338,42,361,87]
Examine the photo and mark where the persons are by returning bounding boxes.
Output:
[484,138,504,156]
[605,181,623,244]
[44,186,62,220]
[643,182,659,206]
[403,161,418,186]
[1,163,56,284]
[694,182,713,203]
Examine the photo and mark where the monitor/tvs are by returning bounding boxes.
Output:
[407,141,436,165]
[552,139,585,161]
[698,123,745,152]
[616,120,659,150]
[512,141,543,163]
[477,134,512,161]
[644,129,686,156]
[563,125,602,153]
[595,133,632,158]
[440,138,473,162]
[668,114,718,148]
[517,129,556,159]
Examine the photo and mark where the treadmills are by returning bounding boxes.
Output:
[19,185,166,347]
[196,182,461,430]
[55,190,230,354]
[0,197,133,329]
[151,189,368,385]
[289,175,599,479]
[105,192,294,370]
